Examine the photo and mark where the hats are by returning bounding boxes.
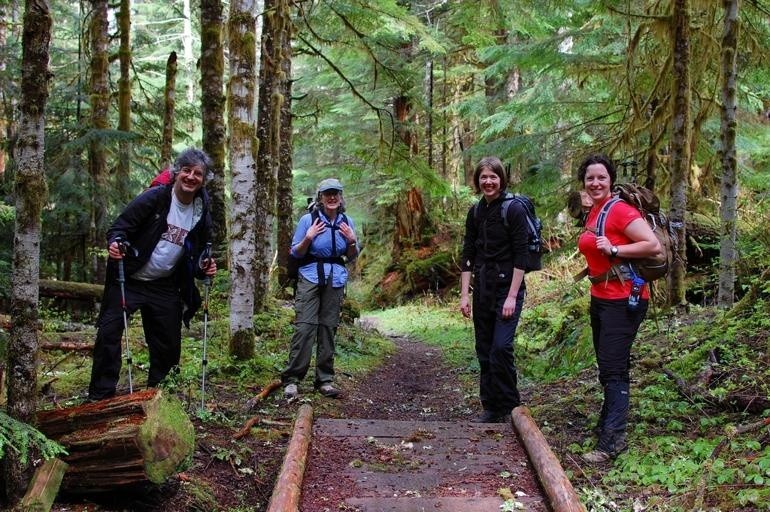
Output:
[319,178,344,192]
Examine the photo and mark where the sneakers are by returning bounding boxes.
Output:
[470,409,513,423]
[581,428,628,463]
[283,383,298,397]
[319,385,341,397]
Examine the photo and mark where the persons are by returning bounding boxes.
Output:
[459,156,529,423]
[577,154,662,465]
[281,178,359,400]
[86,147,218,403]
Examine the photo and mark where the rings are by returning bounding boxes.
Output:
[318,228,320,231]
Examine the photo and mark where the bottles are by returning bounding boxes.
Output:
[627,276,644,311]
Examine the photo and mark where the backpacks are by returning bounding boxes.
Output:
[286,232,302,288]
[612,183,678,283]
[511,194,544,273]
[149,168,176,188]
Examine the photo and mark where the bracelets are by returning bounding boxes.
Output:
[347,240,357,249]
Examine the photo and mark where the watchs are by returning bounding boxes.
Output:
[610,244,618,258]
